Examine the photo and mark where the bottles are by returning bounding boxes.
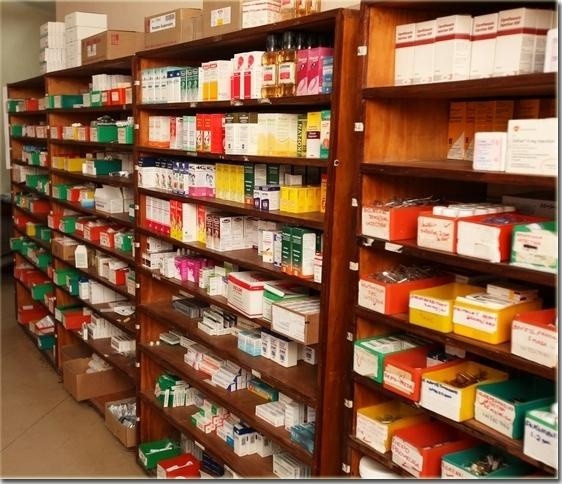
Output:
[260,32,328,99]
